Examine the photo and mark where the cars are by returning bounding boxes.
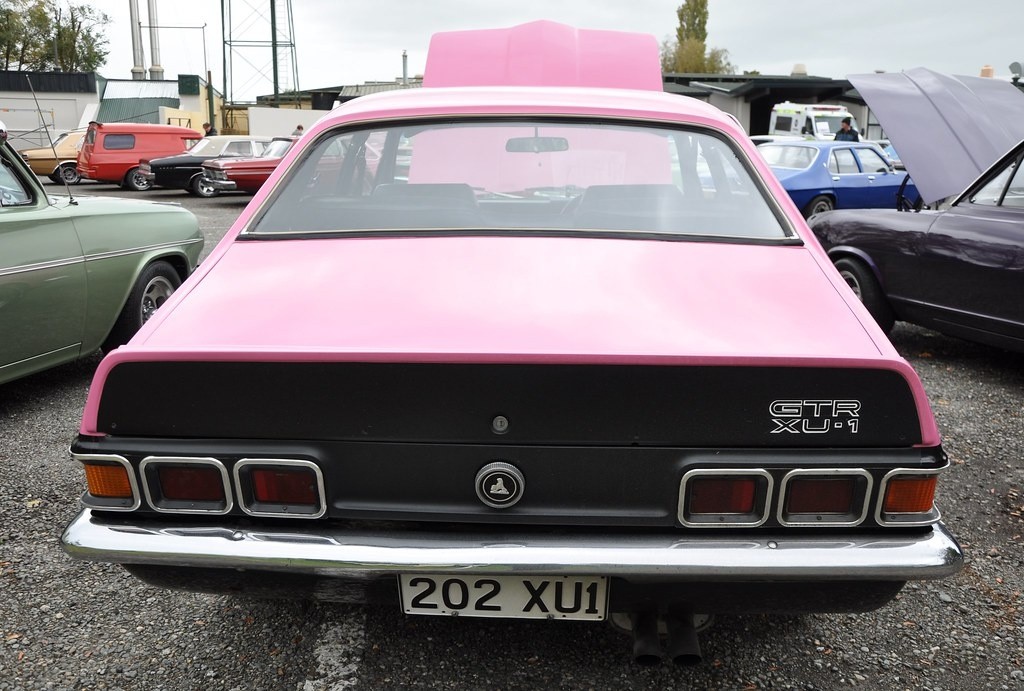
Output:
[198,132,393,200]
[380,145,413,172]
[135,133,273,199]
[16,127,89,187]
[0,127,214,405]
[59,18,969,669]
[803,68,1024,368]
[665,101,927,223]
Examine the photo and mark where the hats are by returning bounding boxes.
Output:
[841,117,851,126]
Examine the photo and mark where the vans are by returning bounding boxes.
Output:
[74,120,209,192]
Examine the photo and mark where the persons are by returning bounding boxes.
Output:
[292,125,303,136]
[203,122,218,137]
[834,117,860,142]
